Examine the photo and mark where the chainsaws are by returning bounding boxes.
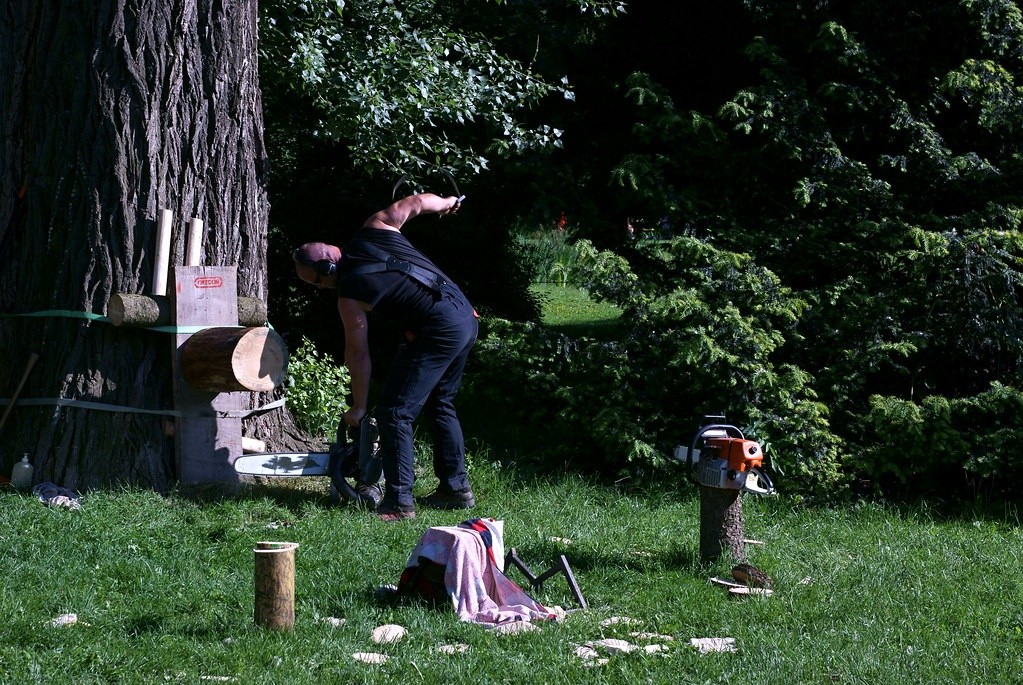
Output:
[231,410,383,508]
[675,414,775,495]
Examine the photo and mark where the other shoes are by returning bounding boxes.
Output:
[372,505,416,521]
[417,487,475,510]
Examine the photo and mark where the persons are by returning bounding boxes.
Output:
[295,193,479,522]
